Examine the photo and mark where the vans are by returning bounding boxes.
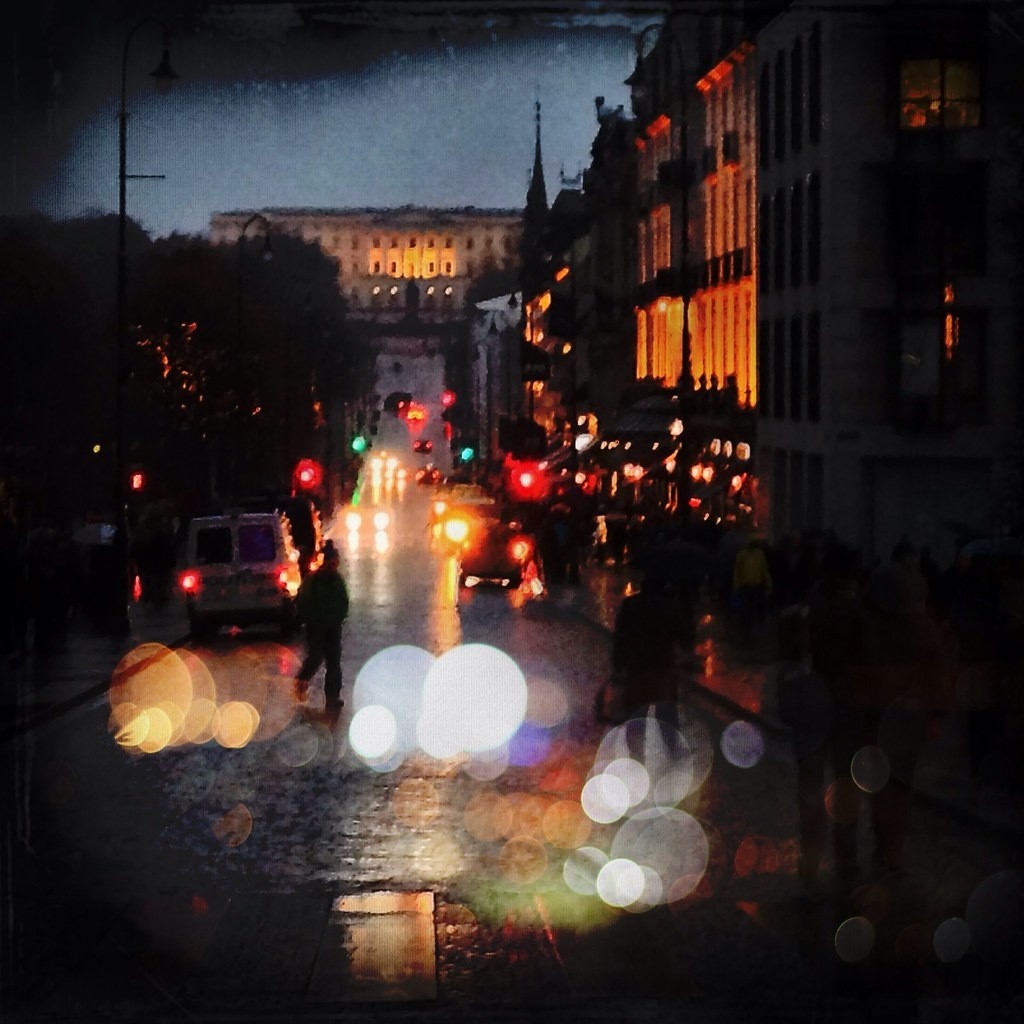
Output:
[187,512,302,640]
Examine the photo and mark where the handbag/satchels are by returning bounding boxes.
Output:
[595,675,633,729]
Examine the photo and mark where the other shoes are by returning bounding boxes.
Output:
[294,678,308,702]
[325,699,344,710]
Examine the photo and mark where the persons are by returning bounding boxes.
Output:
[613,574,698,765]
[777,638,864,878]
[857,622,927,871]
[718,518,822,664]
[293,548,349,708]
